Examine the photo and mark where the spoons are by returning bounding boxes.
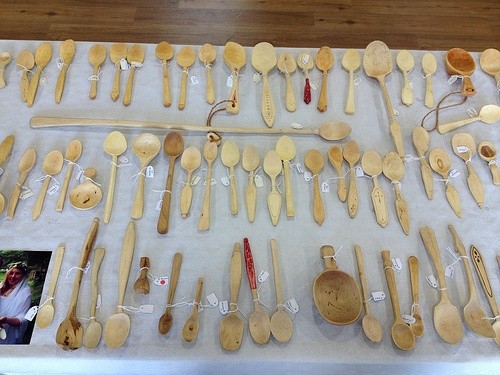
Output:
[104,222,135,348]
[269,240,292,342]
[419,228,462,343]
[380,251,415,351]
[56,219,99,349]
[182,278,203,341]
[408,256,424,337]
[447,225,500,346]
[355,245,383,342]
[243,238,270,344]
[83,249,102,349]
[1,38,500,234]
[159,253,182,335]
[219,242,244,351]
[37,247,64,329]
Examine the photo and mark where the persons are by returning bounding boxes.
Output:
[0,262,32,343]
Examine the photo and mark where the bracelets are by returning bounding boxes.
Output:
[2,316,7,324]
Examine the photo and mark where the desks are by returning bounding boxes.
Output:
[0,0,500,375]
